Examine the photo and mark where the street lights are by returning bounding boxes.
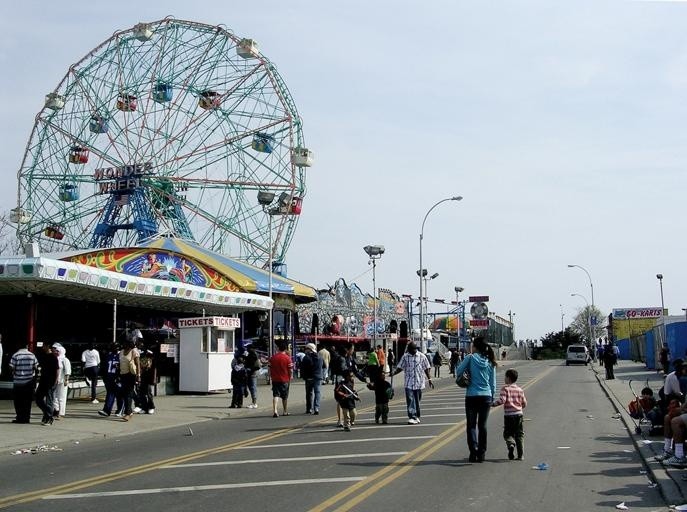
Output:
[257,187,295,382]
[415,268,462,352]
[570,289,592,358]
[361,241,388,351]
[565,261,600,365]
[418,193,464,350]
[654,271,668,352]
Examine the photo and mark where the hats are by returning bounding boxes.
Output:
[305,343,317,353]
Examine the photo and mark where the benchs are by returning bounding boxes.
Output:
[66,380,106,400]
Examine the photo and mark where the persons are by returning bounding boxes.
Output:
[363,370,395,424]
[656,341,671,376]
[330,342,368,427]
[319,320,331,336]
[388,342,437,426]
[330,373,361,430]
[265,341,292,418]
[487,370,526,461]
[229,342,466,408]
[625,359,686,468]
[9,320,156,426]
[298,344,328,414]
[455,336,497,463]
[501,347,506,361]
[330,313,340,335]
[597,336,620,381]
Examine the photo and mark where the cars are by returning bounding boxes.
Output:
[565,344,589,367]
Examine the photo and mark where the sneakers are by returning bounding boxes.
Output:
[507,444,514,459]
[98,410,109,416]
[272,412,278,418]
[407,417,420,424]
[303,408,318,415]
[467,444,485,461]
[114,405,154,421]
[227,402,257,409]
[517,455,523,461]
[283,411,292,416]
[652,450,686,467]
[91,398,99,403]
[40,408,64,426]
[85,377,91,386]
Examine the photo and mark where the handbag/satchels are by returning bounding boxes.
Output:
[455,371,470,388]
[148,367,156,385]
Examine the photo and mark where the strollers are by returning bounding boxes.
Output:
[627,377,658,437]
